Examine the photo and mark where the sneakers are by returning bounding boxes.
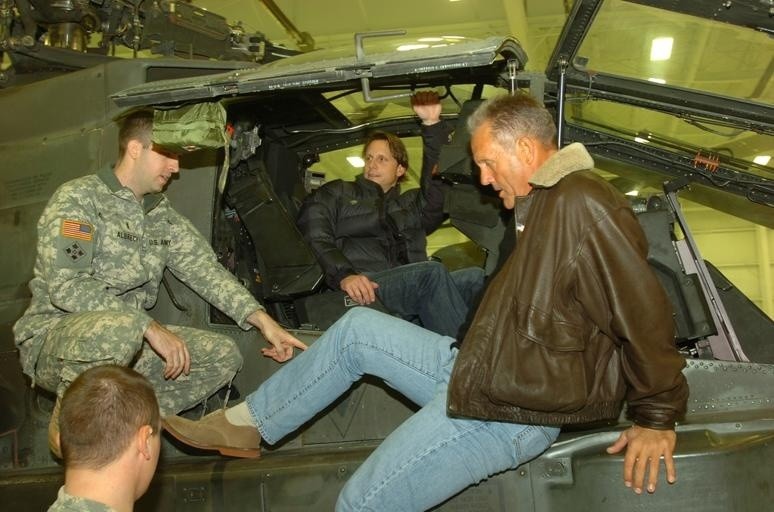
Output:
[162,407,263,458]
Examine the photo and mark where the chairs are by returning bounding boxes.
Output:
[227,139,414,331]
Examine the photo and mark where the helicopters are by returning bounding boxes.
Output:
[0,0,774,511]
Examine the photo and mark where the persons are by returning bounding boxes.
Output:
[159,96,690,511]
[295,90,488,343]
[12,110,309,464]
[43,365,162,510]
